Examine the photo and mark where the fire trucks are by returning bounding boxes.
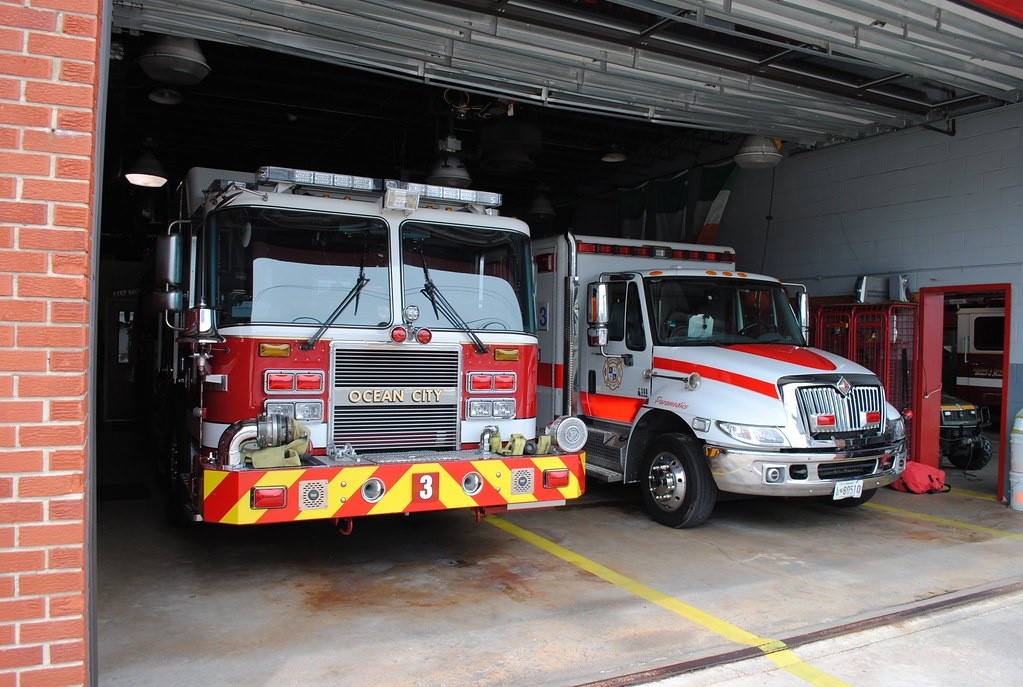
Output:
[101,163,591,538]
[951,308,1007,432]
[472,230,911,532]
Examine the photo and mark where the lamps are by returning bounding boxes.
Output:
[733,135,783,169]
[125,138,168,188]
[147,89,185,105]
[600,142,628,162]
[424,115,473,189]
[135,36,211,86]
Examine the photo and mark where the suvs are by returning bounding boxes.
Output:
[936,394,994,473]
